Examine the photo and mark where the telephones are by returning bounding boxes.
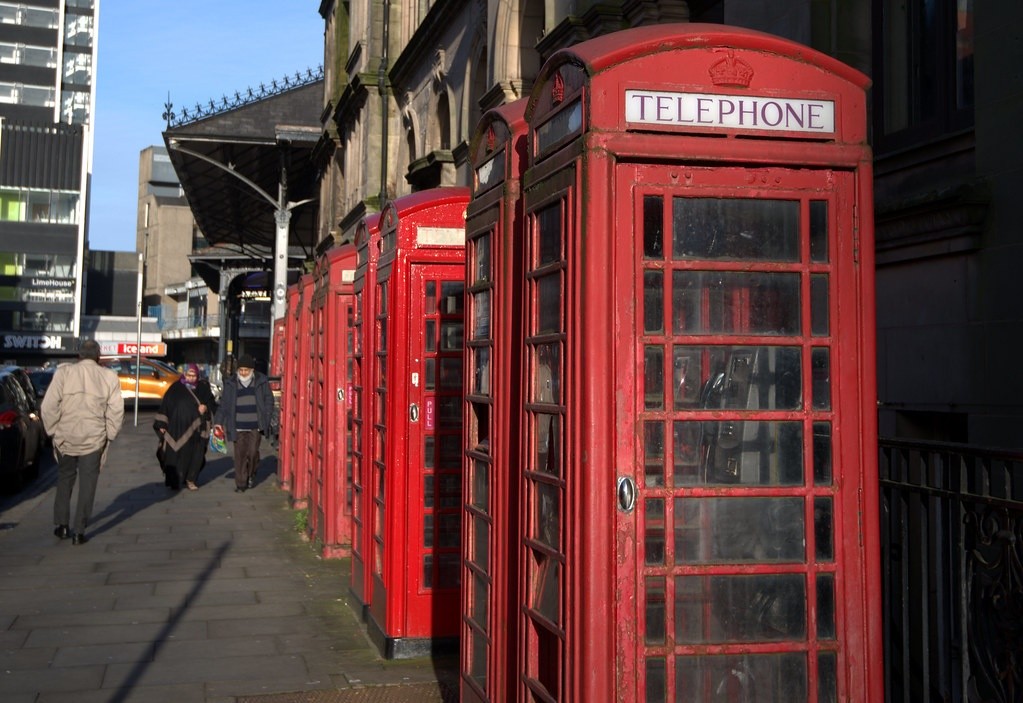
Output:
[698,330,792,485]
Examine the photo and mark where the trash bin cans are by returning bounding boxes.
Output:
[265,375,282,445]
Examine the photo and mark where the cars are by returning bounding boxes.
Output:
[98,355,221,414]
[0,361,61,481]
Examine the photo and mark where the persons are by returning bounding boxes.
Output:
[39,338,124,544]
[213,354,274,493]
[152,362,218,489]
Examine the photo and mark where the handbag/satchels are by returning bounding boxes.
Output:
[208,425,228,455]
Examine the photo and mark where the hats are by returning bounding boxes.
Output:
[236,354,255,371]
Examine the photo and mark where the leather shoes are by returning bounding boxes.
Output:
[54,525,70,539]
[72,533,85,545]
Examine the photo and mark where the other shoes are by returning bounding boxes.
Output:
[186,479,199,492]
[235,488,244,492]
[248,480,254,488]
